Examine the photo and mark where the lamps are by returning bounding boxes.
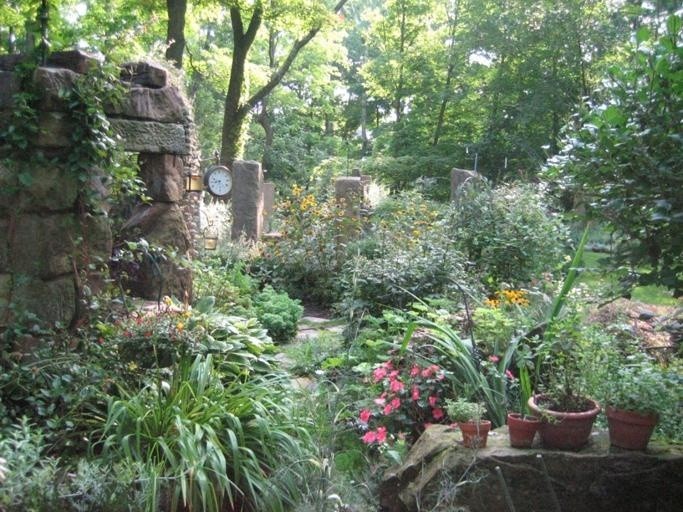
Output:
[184,160,203,194]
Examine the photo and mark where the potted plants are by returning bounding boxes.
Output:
[441,299,680,452]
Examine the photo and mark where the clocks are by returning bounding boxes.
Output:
[205,161,232,197]
